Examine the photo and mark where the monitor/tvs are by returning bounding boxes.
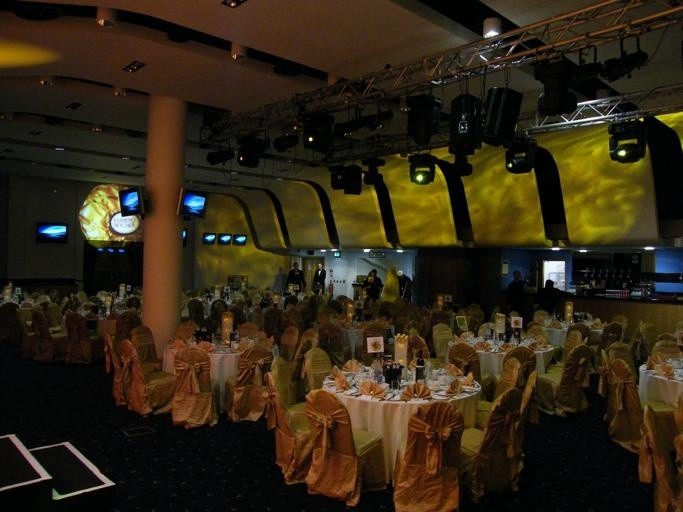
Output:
[120,189,144,217]
[176,187,209,220]
[233,233,247,246]
[218,233,231,245]
[37,224,68,247]
[202,232,216,245]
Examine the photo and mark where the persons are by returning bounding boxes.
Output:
[353,270,384,309]
[0,282,578,320]
[397,270,412,303]
[286,263,307,293]
[506,271,531,309]
[313,263,326,295]
[534,280,573,315]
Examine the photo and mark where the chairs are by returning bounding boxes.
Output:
[0,292,683,512]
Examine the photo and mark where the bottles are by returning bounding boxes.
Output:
[490,325,520,344]
[191,325,240,355]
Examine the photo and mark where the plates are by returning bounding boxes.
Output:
[638,358,682,382]
[324,351,479,403]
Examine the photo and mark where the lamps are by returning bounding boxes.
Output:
[448,78,482,157]
[407,151,437,186]
[403,90,444,147]
[532,36,649,116]
[208,138,235,167]
[301,111,336,149]
[608,119,648,164]
[483,68,524,148]
[273,126,299,153]
[235,129,267,169]
[328,160,363,195]
[504,137,538,175]
